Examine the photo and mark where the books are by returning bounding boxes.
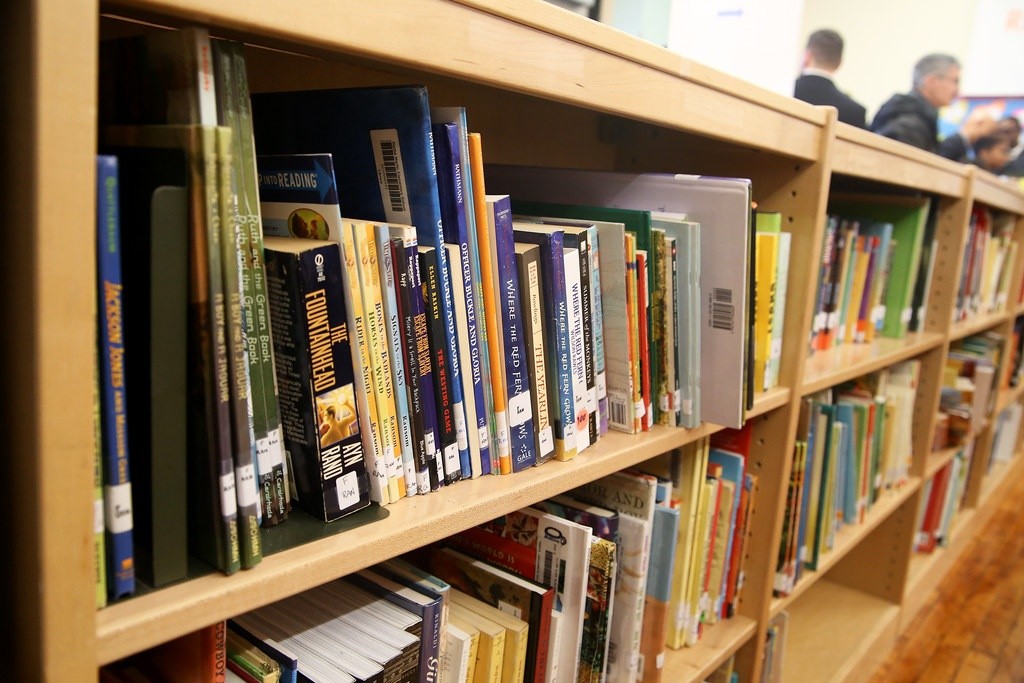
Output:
[96,22,1024,683]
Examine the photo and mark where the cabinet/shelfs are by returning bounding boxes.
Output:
[0,0,1024,683]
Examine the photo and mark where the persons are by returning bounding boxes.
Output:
[1000,116,1024,177]
[794,29,865,130]
[871,53,997,162]
[967,117,1010,190]
